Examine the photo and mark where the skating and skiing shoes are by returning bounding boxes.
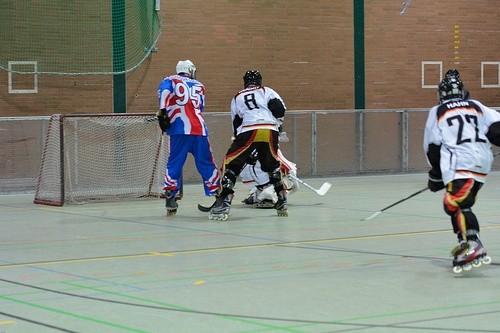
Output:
[208,194,235,221]
[275,200,289,217]
[166,196,179,216]
[452,239,491,273]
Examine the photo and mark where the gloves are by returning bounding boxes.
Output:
[156,111,170,132]
[428,170,445,193]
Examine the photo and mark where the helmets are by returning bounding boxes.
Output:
[176,59,197,78]
[244,70,262,89]
[438,69,470,103]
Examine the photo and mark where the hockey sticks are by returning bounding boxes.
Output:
[284,173,333,196]
[360,151,500,221]
[197,200,217,212]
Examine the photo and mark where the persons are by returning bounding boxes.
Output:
[423,68,500,267]
[156,60,222,211]
[240,148,299,205]
[208,70,289,215]
